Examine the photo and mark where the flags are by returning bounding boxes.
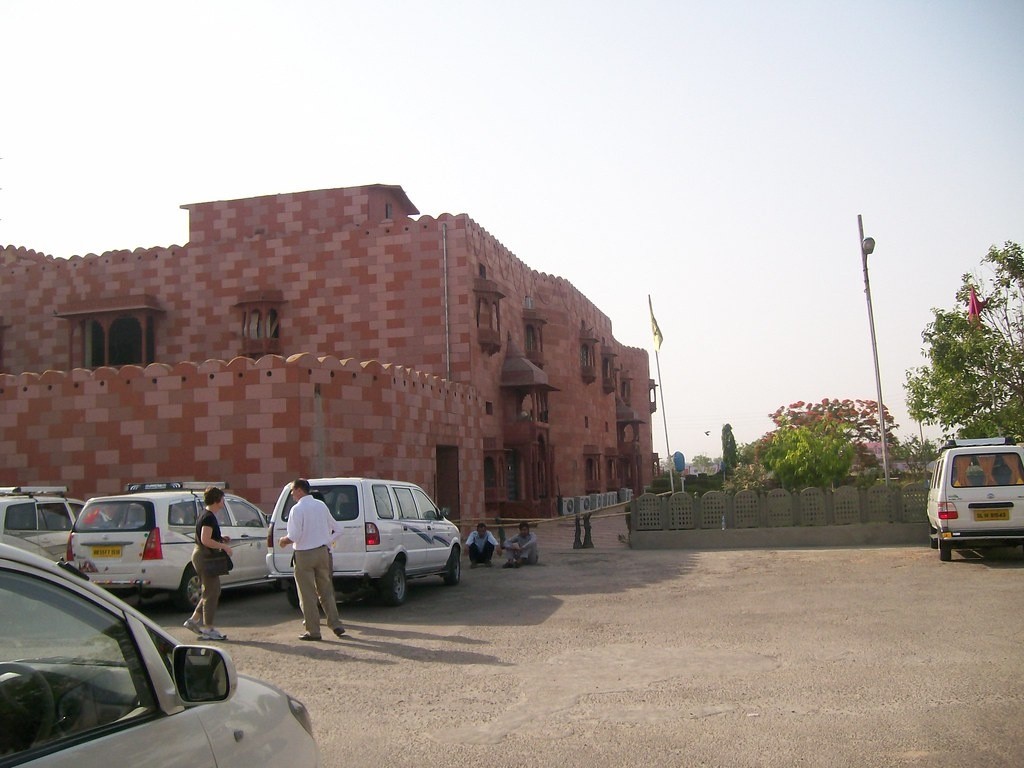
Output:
[968,288,990,330]
[649,301,663,351]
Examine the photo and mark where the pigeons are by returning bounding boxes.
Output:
[722,515,727,530]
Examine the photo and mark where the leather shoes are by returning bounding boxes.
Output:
[333,628,345,636]
[299,633,321,641]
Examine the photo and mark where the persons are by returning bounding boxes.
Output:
[279,478,346,641]
[183,486,233,640]
[517,409,537,421]
[465,523,502,569]
[502,523,538,569]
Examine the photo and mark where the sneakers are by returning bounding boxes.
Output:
[202,629,226,640]
[183,618,203,635]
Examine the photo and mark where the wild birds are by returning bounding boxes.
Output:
[704,431,710,437]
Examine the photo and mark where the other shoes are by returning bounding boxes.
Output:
[509,562,520,568]
[502,562,511,568]
[471,563,477,568]
[485,561,491,567]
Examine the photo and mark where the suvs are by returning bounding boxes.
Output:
[0,486,119,568]
[65,481,276,614]
[264,477,462,608]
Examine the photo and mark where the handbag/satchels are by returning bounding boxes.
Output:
[203,551,233,575]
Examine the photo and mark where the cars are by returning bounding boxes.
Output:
[0,544,321,768]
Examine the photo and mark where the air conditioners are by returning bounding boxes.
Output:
[558,487,633,517]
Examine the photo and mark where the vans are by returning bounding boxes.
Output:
[926,438,1024,562]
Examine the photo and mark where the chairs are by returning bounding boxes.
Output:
[339,503,357,520]
[46,513,66,530]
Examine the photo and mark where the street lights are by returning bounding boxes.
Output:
[858,214,891,485]
